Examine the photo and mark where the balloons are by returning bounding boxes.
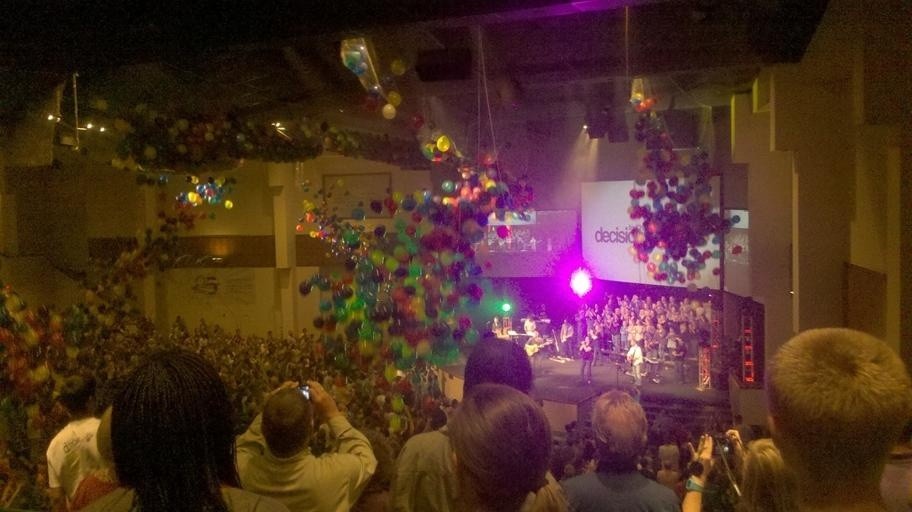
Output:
[290,138,536,298]
[312,290,484,430]
[338,38,446,138]
[83,90,371,188]
[590,76,747,291]
[76,188,236,293]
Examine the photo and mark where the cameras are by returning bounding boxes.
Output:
[714,435,733,458]
[296,383,312,401]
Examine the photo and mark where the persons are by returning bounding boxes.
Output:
[0,339,794,512]
[489,292,712,384]
[766,329,911,511]
[0,292,311,349]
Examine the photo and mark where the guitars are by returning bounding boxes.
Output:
[525,342,553,356]
